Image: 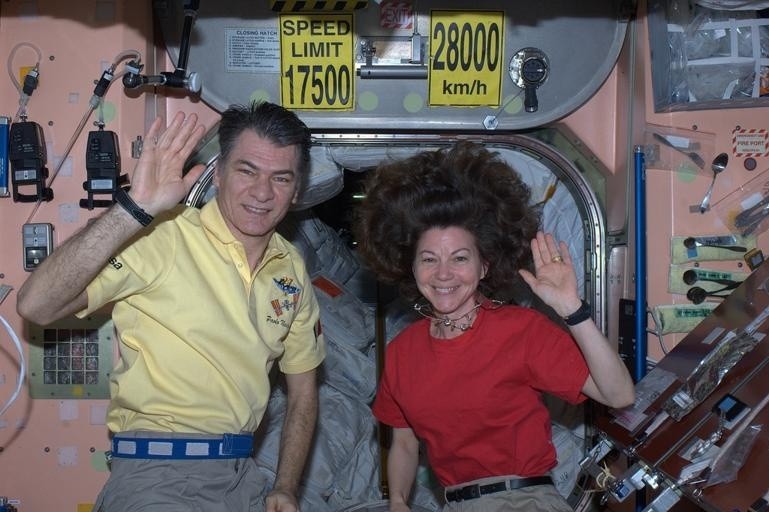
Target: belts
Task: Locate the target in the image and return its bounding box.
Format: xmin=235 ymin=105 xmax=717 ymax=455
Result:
xmin=110 ymin=432 xmax=256 ymax=461
xmin=444 ymin=475 xmax=554 ymax=504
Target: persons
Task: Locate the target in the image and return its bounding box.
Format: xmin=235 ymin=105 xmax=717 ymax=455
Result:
xmin=347 ymin=139 xmax=641 ymax=511
xmin=15 ymin=97 xmax=331 ymax=512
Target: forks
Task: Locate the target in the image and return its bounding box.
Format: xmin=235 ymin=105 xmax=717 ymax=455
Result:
xmin=654 ymin=133 xmax=706 ymax=169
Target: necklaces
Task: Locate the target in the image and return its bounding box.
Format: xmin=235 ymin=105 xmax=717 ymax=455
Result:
xmin=413 ymin=298 xmax=505 ymax=331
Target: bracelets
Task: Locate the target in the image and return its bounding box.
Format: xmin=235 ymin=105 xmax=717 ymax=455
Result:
xmin=560 ymin=298 xmax=592 ymax=326
xmin=113 ymin=189 xmax=154 ymax=229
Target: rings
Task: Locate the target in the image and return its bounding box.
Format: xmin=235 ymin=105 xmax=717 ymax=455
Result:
xmin=551 ymin=255 xmax=562 ymax=264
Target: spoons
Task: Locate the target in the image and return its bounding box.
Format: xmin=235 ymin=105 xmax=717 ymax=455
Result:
xmin=683 ymin=270 xmax=743 ymax=305
xmin=683 ymin=237 xmax=747 ymax=252
xmin=699 ymin=153 xmax=729 ymax=215
